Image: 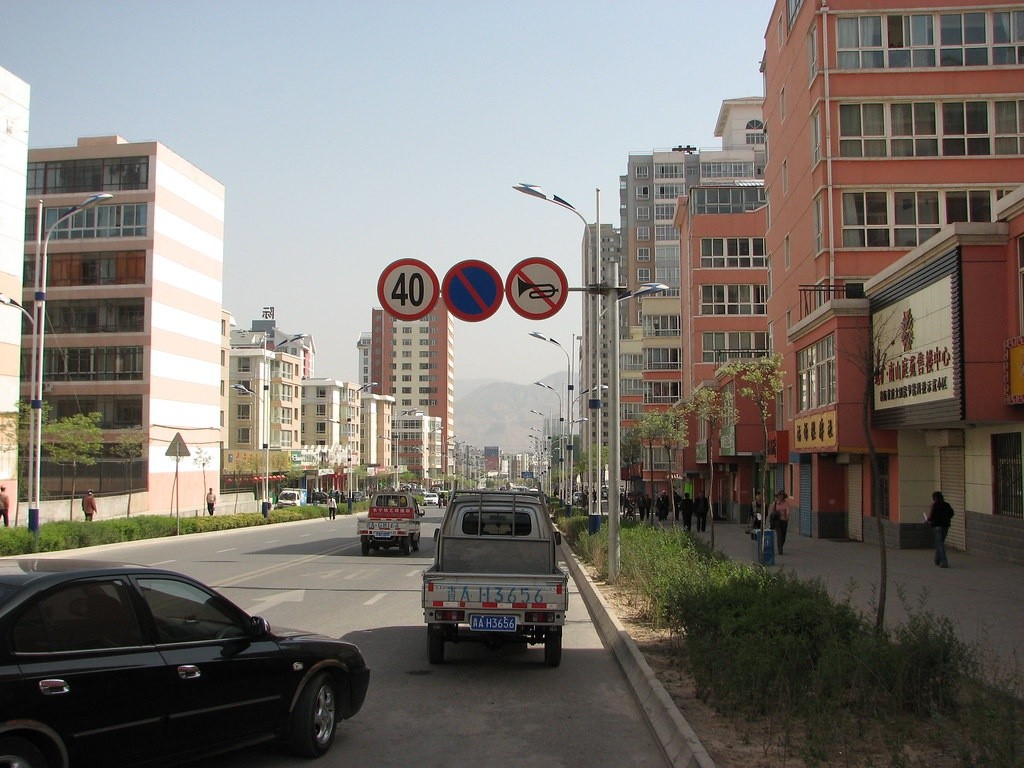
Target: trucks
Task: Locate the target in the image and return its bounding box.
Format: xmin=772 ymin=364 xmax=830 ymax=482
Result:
xmin=356 ymin=489 xmax=426 ymax=558
xmin=420 ymin=479 xmax=571 ymax=669
xmin=275 ymin=487 xmax=308 ymax=509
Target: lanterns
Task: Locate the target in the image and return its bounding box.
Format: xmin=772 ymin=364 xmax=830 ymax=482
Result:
xmin=226 ymin=475 xmax=285 ymax=484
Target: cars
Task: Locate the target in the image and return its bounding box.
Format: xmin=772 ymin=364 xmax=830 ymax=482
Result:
xmin=0 ymin=557 xmax=373 ymax=768
xmin=422 ymin=492 xmax=439 ymax=506
xmin=572 ymin=491 xmax=583 ymax=505
xmin=349 ymin=490 xmax=365 ymax=501
xmin=307 ymin=491 xmax=329 ymax=504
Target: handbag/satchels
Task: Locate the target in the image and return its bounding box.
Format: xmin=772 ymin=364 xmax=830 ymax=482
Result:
xmin=770 ymin=510 xmax=779 ymax=529
xmin=745 ymin=524 xmax=753 ymax=533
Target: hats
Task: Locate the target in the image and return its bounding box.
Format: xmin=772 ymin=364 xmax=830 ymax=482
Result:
xmin=775 ymin=489 xmax=786 ymax=498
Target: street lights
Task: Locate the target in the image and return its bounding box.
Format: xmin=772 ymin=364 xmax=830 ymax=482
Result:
xmin=0 ymin=193 xmax=118 ymax=536
xmin=229 ymin=333 xmax=310 ymax=517
xmin=324 ymin=381 xmax=379 ymax=514
xmin=526 ymin=427 xmax=562 ymax=495
xmin=377 ymin=408 xmax=418 ymax=493
xmin=512 ymin=182 xmax=671 ymax=535
xmin=529 ymin=408 xmax=568 ymax=505
xmin=527 ymin=330 xmax=609 ymax=518
xmin=533 ymin=379 xmax=589 ymax=507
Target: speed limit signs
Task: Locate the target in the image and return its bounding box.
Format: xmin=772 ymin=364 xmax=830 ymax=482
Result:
xmin=377 ymin=257 xmax=440 ymax=322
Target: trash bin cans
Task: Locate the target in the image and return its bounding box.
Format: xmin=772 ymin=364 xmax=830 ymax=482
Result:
xmin=751 ymin=529 xmax=775 ymax=566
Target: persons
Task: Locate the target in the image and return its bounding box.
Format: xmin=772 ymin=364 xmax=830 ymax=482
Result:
xmin=656 ymin=490 xmax=669 ymax=521
xmin=82 ymin=489 xmax=97 ymax=521
xmin=637 ymin=492 xmax=652 ymax=521
xmin=0 ymin=485 xmax=10 ymax=527
xmin=925 ymin=492 xmax=954 ymax=568
xmin=438 ymin=497 xmax=443 ymax=508
xmin=554 ymin=489 xmax=559 ymax=497
xmin=620 ymin=493 xmax=624 ymax=512
xmin=206 ymin=488 xmax=216 ymax=515
xmin=334 ymin=491 xmax=345 ymax=503
xmin=694 ymin=490 xmax=708 ymax=532
xmin=771 ymin=489 xmax=791 ymax=554
xmin=674 ymin=492 xmax=682 ymax=521
xmin=751 ymin=492 xmax=761 ymax=540
xmin=680 ymin=492 xmax=694 ymax=531
xmin=328 ymin=496 xmax=337 ymax=520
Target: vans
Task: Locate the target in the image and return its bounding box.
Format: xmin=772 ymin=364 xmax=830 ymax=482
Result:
xmin=601 ymin=484 xmax=625 ymax=499
